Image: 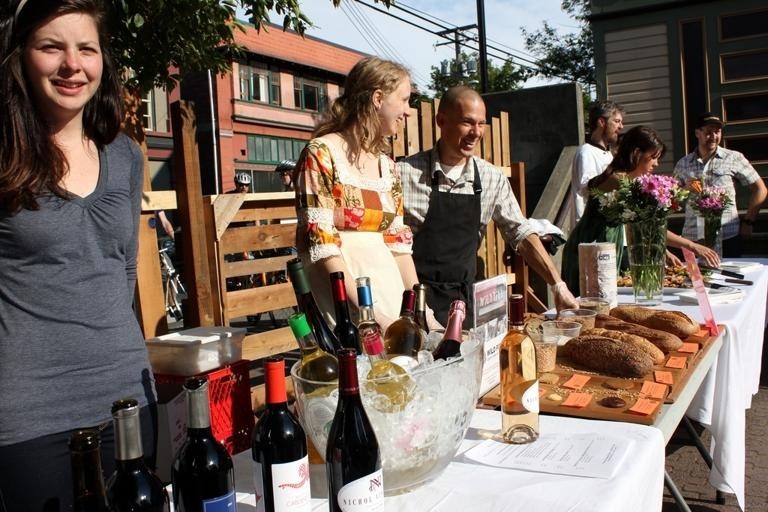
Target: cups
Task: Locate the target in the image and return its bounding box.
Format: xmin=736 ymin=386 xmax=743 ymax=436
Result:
xmin=527 ymin=298 xmax=610 ymax=372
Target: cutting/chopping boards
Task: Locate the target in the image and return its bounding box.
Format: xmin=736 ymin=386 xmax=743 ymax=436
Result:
xmin=482 ymin=322 xmax=725 ymax=425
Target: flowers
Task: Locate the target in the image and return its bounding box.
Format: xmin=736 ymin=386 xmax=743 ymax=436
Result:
xmin=581 ymin=170 xmax=690 ymax=300
xmin=680 ymin=174 xmax=734 ymax=258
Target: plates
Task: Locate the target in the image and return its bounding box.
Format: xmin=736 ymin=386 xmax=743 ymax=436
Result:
xmin=617 ymin=284 xmax=692 ymax=295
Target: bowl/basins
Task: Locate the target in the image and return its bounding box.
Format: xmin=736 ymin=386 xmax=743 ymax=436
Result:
xmin=287 ymin=331 xmax=483 ymax=498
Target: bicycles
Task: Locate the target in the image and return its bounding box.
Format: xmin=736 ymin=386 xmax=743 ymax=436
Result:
xmin=159 ymin=242 xmax=188 ymax=323
xmin=234 ymin=225 xmax=299 ymax=331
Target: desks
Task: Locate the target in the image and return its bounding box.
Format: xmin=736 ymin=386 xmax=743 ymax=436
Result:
xmin=539 ymin=251 xmax=768 ymax=506
xmin=167 ymin=310 xmax=728 ymax=512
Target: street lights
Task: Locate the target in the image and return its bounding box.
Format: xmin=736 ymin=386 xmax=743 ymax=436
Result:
xmin=438 ymin=56 xmax=480 ymax=91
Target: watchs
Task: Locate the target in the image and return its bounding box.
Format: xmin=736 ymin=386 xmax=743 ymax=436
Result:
xmin=744 ymin=217 xmax=755 ymax=227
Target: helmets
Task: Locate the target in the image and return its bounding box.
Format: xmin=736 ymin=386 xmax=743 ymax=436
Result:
xmin=234 ymin=170 xmax=252 ymax=185
xmin=274 ymin=159 xmax=297 ymax=172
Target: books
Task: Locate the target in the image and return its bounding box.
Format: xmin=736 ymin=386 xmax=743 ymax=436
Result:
xmin=681 ymin=287 xmax=744 ymax=303
xmin=719 ymin=260 xmax=763 ymax=271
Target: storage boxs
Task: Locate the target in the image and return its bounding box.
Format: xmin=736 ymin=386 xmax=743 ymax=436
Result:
xmin=152 ymin=361 xmax=257 ymax=459
xmin=139 ymin=323 xmax=249 ymax=375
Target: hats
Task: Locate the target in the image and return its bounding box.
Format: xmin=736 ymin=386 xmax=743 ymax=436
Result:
xmin=695 ymin=112 xmax=724 ymax=129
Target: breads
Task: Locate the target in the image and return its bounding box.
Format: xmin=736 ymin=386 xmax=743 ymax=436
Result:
xmin=564 ymin=306 xmax=701 ymax=378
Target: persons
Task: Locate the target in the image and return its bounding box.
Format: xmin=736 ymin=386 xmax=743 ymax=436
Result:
xmin=221 ymin=171 xmax=266 ymax=289
xmin=273 ymin=158 xmax=300 ymax=282
xmin=404 ymin=86 xmax=584 ymax=328
xmin=558 ymin=125 xmax=721 ymax=297
xmin=1 ymin=2 xmax=161 ymax=509
xmin=156 ymin=208 xmax=176 ymax=318
xmin=569 ymin=99 xmax=625 ymax=235
xmin=671 ymin=112 xmax=768 ymax=258
xmin=292 ymin=57 xmax=444 ymax=338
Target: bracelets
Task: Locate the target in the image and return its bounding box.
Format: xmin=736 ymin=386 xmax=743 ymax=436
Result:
xmin=551 ymin=281 xmax=570 ymax=295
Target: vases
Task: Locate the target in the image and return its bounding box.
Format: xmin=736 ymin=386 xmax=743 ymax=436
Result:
xmin=620 ymin=212 xmax=669 ymax=305
xmin=703 ymin=215 xmax=722 ymax=267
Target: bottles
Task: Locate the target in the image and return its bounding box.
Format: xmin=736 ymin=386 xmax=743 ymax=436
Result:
xmin=324 ymin=349 xmax=386 ymax=511
xmin=104 ymin=397 xmax=171 ymax=511
xmin=499 ymin=294 xmax=541 ymax=445
xmin=172 ymin=378 xmax=236 ymax=510
xmin=250 ymin=358 xmax=313 ymax=512
xmin=285 ymin=256 xmax=469 ymax=466
xmin=71 ymin=431 xmax=110 ymax=505
